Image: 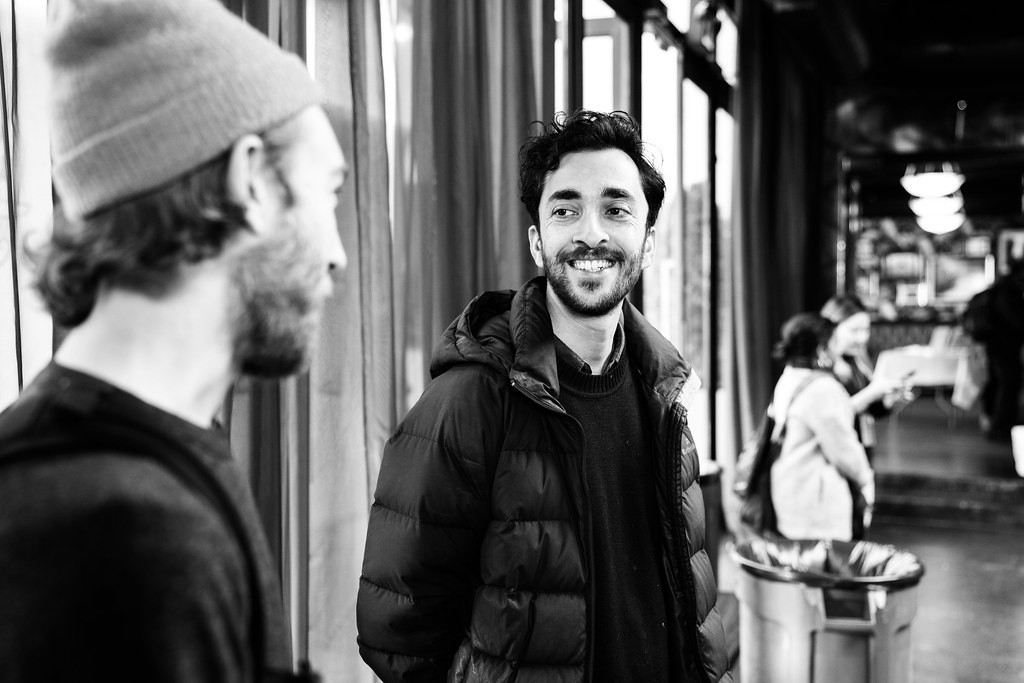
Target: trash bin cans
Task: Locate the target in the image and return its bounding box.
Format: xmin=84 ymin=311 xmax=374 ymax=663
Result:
xmin=734 ymin=539 xmax=925 ymax=683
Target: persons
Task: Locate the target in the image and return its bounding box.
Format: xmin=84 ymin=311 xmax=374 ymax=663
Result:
xmin=766 ymin=314 xmax=878 ymax=543
xmin=353 ymin=110 xmax=738 ymax=683
xmin=0 ymin=1 xmax=348 ymax=683
xmin=817 ymin=292 xmax=917 ymax=542
xmin=964 ymin=256 xmax=1023 ymax=442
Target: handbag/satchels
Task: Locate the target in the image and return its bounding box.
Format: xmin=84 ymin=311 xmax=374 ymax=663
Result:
xmin=731 ymin=410 xmax=776 ymax=503
xmin=739 ymin=443 xmax=782 ymax=538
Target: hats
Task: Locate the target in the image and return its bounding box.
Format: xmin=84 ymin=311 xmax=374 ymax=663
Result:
xmin=47 ymin=0 xmax=324 ymax=221
